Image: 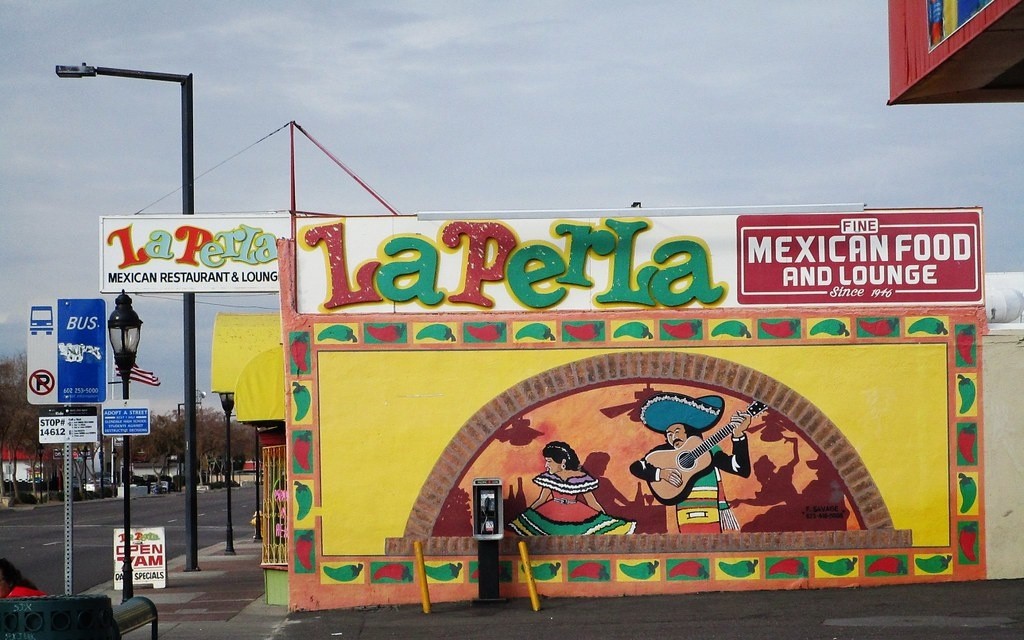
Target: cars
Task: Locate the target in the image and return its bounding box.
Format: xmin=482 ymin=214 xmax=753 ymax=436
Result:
xmin=5 ymin=471 xmax=172 ymax=497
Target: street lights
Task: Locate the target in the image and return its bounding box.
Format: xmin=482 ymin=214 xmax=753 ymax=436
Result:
xmin=108 ymin=289 xmax=144 ymax=606
xmin=217 ymin=392 xmax=236 ymax=557
xmin=56 ymin=60 xmax=198 ymax=570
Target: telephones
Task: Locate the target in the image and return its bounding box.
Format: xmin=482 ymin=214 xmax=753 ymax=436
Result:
xmin=481 ymin=497 xmax=495 ymax=511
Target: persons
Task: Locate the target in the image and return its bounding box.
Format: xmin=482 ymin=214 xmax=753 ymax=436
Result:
xmin=0 ymin=557 xmax=47 ymax=598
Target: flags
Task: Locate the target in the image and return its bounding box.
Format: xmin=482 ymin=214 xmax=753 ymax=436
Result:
xmin=113 ymin=352 xmax=161 ymax=386
